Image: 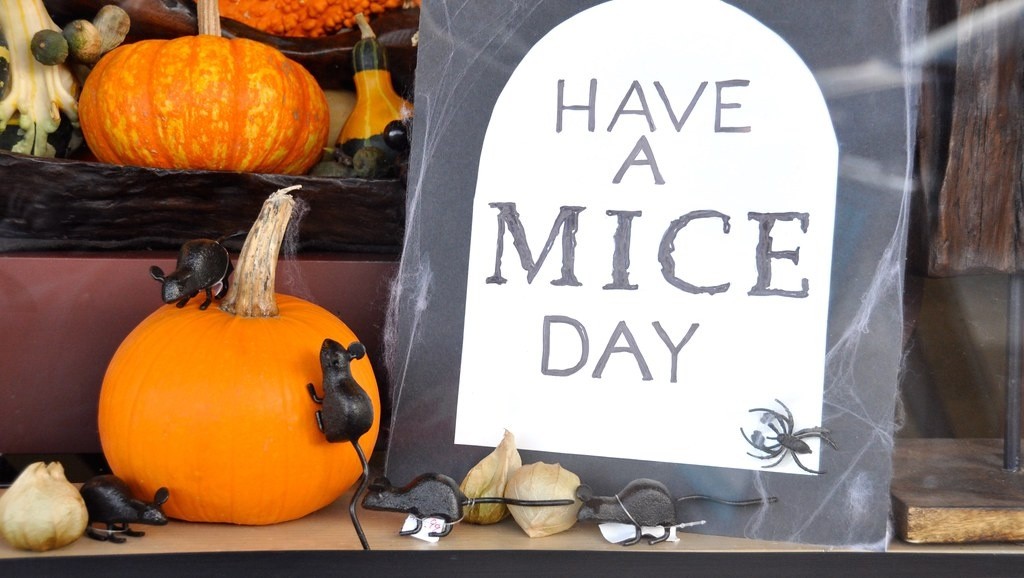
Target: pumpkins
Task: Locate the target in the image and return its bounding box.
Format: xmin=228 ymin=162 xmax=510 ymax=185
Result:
xmin=97 ymin=184 xmax=380 ymax=526
xmin=0 ymin=0 xmax=423 ymax=177
